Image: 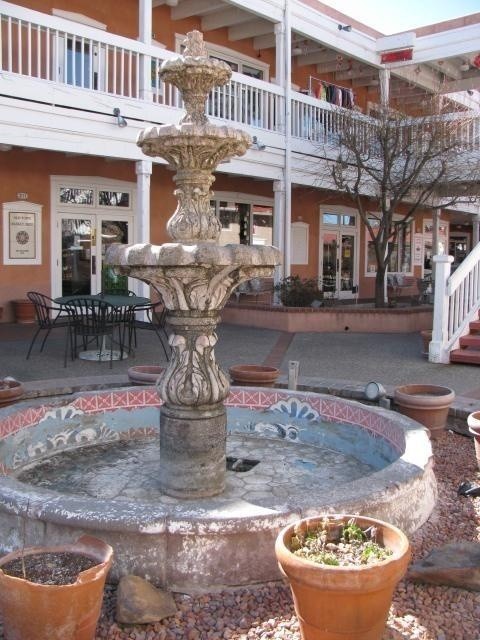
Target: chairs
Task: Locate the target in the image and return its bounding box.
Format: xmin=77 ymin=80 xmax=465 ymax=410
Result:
xmin=21 ymin=286 xmax=176 ymax=368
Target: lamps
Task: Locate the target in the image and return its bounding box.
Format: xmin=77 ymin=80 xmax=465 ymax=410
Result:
xmin=363 ymin=380 xmax=393 ymax=410
xmin=249 ymin=133 xmax=269 ymax=154
xmin=109 ymin=105 xmax=131 ymax=130
xmin=336 ymin=20 xmax=354 ymax=36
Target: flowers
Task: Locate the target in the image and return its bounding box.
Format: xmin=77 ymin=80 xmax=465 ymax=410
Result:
xmin=271 ymin=272 xmax=321 ymax=294
xmin=287 ymin=512 xmax=388 ymax=567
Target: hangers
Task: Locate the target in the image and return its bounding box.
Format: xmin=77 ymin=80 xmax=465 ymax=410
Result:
xmin=314 ymin=79 xmax=354 ymax=92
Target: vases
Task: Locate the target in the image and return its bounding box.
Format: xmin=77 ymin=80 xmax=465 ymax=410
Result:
xmin=279 ymin=287 xmax=315 ymax=308
xmin=12 ymin=298 xmax=39 ymax=327
xmin=0 ymin=378 xmax=27 ymax=411
xmin=228 ymin=365 xmax=281 ymax=389
xmin=0 ymin=533 xmax=117 ymax=640
xmin=466 ymin=409 xmax=480 ymax=471
xmin=391 ymin=382 xmax=457 ymax=440
xmin=127 ymin=363 xmax=168 ymax=387
xmin=273 ymin=511 xmax=413 ymax=640
